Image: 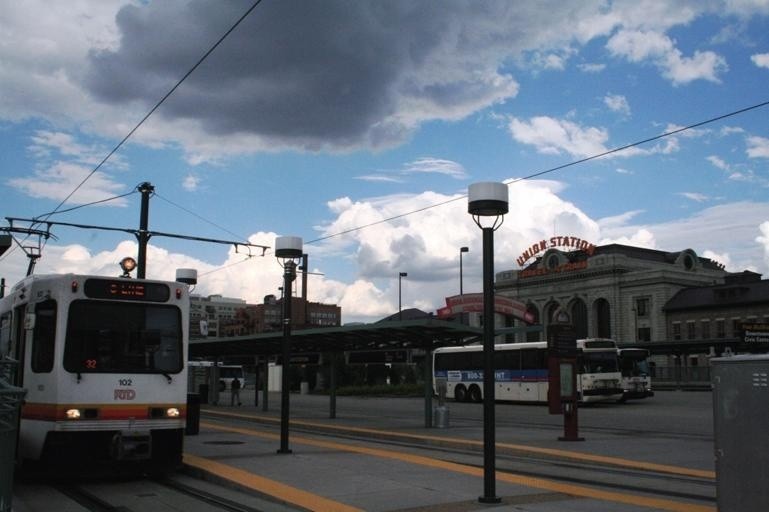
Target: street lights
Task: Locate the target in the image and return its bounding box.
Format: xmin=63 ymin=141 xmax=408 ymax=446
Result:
xmin=399 ymin=272 xmax=407 ymax=321
xmin=460 ymin=246 xmax=469 ymax=295
xmin=275 ymin=235 xmax=302 ymax=453
xmin=467 ymin=182 xmax=509 ymax=505
xmin=278 ymin=287 xmax=283 ymax=330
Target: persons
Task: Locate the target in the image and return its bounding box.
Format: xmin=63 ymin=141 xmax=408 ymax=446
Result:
xmin=230 ymin=376 xmax=242 ymax=407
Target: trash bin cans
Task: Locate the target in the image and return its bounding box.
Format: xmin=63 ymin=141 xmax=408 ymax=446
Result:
xmin=434 ymin=406 xmax=449 ymax=428
xmin=186 ymin=392 xmax=200 ymax=434
xmin=199 ymin=384 xmax=208 ymax=404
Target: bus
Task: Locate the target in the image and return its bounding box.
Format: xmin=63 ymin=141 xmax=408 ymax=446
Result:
xmin=431 ymin=337 xmax=654 ymax=405
xmin=188 ymin=360 xmax=247 ymax=392
xmin=0 ymin=258 xmax=189 ymax=476
